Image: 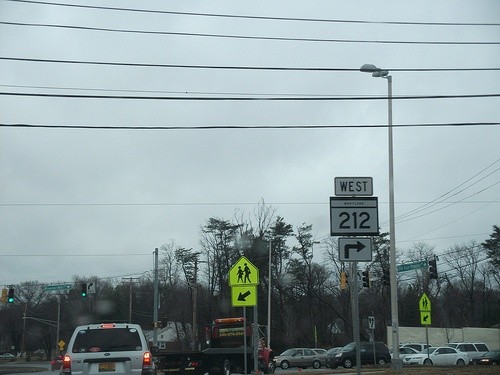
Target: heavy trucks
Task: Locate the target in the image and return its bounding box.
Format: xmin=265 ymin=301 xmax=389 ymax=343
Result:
xmin=153 ymin=317 xmax=276 ymax=375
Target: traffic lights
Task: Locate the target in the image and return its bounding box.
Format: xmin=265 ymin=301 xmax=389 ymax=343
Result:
xmin=81 ymin=284 xmax=87 ymax=297
xmin=429 ymin=259 xmax=437 ymax=278
xmin=9 ymin=289 xmax=14 ymax=303
xmin=362 ymin=271 xmax=371 ymax=288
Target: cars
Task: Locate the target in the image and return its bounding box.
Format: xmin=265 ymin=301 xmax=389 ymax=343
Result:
xmin=398 ymin=343 xmax=432 ymax=351
xmin=446 ymin=342 xmax=491 ymax=361
xmin=315 ymin=347 xmax=342 ymax=368
xmin=399 ymin=347 xmax=420 ymax=361
xmin=469 ymin=350 xmax=500 ymax=366
xmin=404 ymin=347 xmax=469 ymax=367
xmin=274 ymin=348 xmax=326 ymax=370
xmin=51 ymin=356 xmax=63 ymax=371
xmin=325 ymin=341 xmax=391 ymax=368
xmin=59 ymin=323 xmax=157 ymax=375
xmin=0 ymin=353 xmax=14 ymax=360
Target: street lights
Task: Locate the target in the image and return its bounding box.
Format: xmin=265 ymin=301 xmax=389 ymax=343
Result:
xmin=359 ymin=64 xmax=399 ymax=358
